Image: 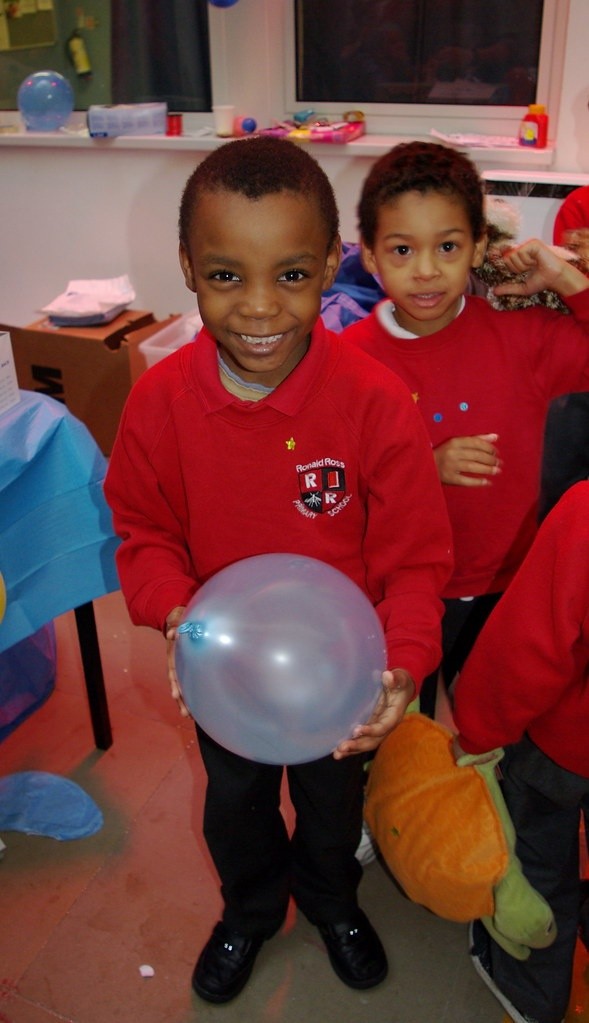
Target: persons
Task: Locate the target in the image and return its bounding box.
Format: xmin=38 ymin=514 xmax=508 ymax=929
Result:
xmin=102 ymin=136 xmax=456 ymax=1002
xmin=553 ymin=186 xmax=589 ymax=248
xmin=453 ymin=480 xmax=589 ymax=1023
xmin=342 ymin=142 xmax=588 ymax=868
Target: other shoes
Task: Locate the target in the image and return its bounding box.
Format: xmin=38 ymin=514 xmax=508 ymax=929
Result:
xmin=468 ymin=919 xmax=561 ymax=1023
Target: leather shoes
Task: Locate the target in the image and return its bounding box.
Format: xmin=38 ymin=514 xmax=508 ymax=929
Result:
xmin=191 ymin=920 xmax=284 ymax=1006
xmin=318 ymin=905 xmax=389 ymax=990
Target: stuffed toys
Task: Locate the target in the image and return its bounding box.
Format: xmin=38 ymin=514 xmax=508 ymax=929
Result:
xmin=473 ymin=198 xmax=568 ymax=314
xmin=364 ymin=696 xmax=557 ymax=959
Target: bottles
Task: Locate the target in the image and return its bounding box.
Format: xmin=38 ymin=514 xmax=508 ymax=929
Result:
xmin=519 ymin=104 xmax=549 ymax=149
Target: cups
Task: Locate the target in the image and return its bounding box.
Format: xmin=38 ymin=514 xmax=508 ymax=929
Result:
xmin=212 ymin=105 xmax=235 ymax=138
xmin=166 ymin=114 xmax=183 ymax=137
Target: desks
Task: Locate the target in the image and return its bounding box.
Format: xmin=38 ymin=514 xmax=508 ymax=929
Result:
xmin=0 ymin=391 xmax=112 ymax=752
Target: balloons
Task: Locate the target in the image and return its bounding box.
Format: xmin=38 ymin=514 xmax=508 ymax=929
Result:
xmin=17 ymin=71 xmax=75 ymax=132
xmin=174 ymin=553 xmax=386 ymax=762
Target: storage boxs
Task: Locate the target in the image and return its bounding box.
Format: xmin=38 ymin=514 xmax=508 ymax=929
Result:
xmin=87 ymin=103 xmax=167 ymax=138
xmin=0 ymin=307 xmax=204 ymax=457
